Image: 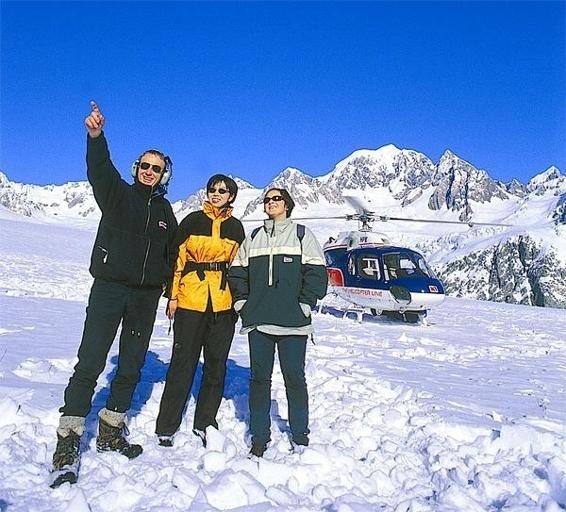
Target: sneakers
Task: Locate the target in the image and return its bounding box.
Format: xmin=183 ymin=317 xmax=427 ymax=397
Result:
xmin=249 ymin=443 xmax=269 ymax=459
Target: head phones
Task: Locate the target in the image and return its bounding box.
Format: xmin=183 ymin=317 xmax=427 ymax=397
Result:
xmin=131 ymin=150 xmax=173 ymax=186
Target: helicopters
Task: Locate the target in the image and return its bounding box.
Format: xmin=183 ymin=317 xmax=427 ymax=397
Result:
xmin=239 ymin=208 xmax=513 ymax=327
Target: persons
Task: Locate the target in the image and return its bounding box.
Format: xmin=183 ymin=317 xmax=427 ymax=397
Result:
xmin=226 ymin=187 xmax=328 ymax=463
xmin=154 ymin=173 xmax=246 ymax=454
xmin=46 ymin=99 xmax=179 ymax=492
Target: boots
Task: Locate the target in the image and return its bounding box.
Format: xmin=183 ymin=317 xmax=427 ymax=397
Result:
xmin=94 ymin=405 xmax=142 ymax=461
xmin=46 ymin=414 xmax=88 ymax=489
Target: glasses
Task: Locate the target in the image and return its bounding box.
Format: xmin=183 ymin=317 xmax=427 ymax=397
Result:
xmin=263 ymin=196 xmax=283 ymax=204
xmin=138 ymin=162 xmax=164 ymax=174
xmin=207 ymin=187 xmax=230 ymax=195
